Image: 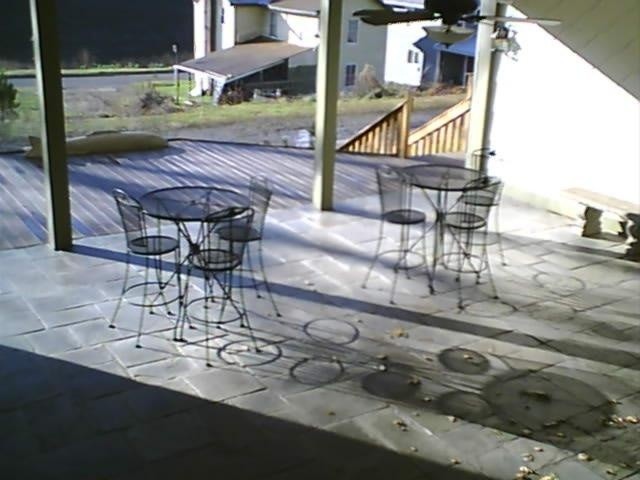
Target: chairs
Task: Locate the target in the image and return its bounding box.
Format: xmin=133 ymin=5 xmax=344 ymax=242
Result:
xmin=106 ymin=174 xmax=285 ymax=369
xmin=361 ymin=165 xmax=508 ymax=301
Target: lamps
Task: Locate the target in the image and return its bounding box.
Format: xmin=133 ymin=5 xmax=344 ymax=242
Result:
xmin=420 ymin=1 xmax=481 ymax=48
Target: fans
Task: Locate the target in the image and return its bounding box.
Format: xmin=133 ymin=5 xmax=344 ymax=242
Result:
xmin=352 ymin=1 xmax=563 ymax=49
